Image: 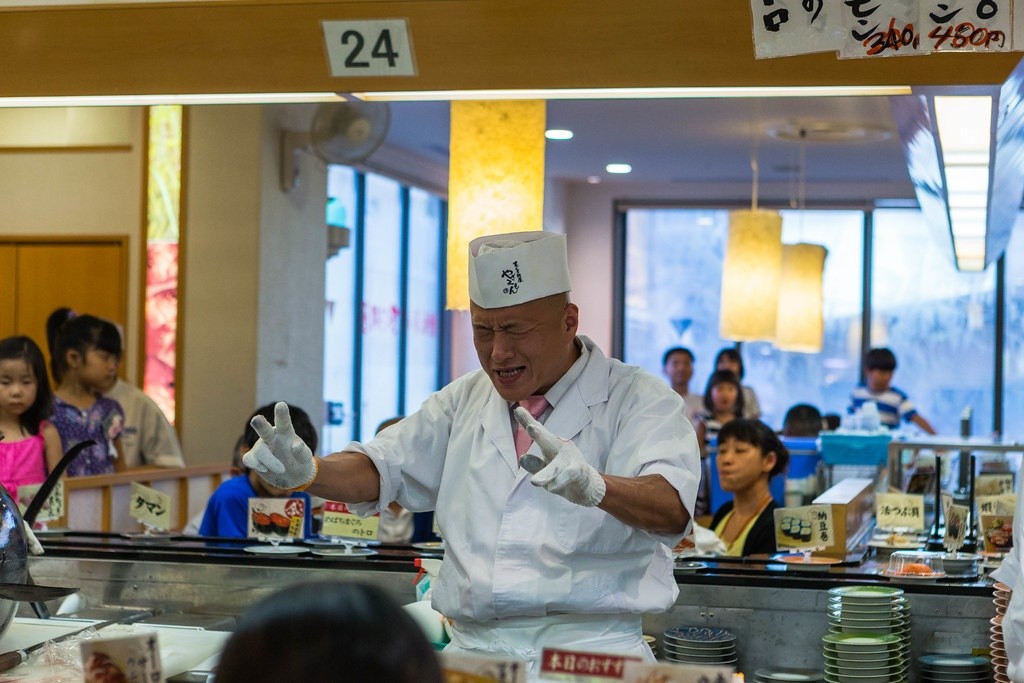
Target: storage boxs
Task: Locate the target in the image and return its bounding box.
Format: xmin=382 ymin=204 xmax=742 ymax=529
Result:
xmin=817 ymin=429 xmax=912 ymax=467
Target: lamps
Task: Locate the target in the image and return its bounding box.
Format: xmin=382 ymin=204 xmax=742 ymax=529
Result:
xmin=717 ymin=98 xmax=782 ymax=340
xmin=444 ymin=96 xmax=547 ymax=315
xmin=775 ymin=126 xmax=828 ymax=353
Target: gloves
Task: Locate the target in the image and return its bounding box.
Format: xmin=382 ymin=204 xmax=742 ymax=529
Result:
xmin=241 ymin=401 xmax=320 ymax=493
xmin=513 ymin=405 xmax=607 ymax=508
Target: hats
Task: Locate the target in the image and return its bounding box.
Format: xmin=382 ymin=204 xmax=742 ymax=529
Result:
xmin=468 ymin=230 xmax=571 ymax=310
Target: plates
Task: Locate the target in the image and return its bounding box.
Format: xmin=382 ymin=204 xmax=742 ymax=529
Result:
xmin=775 ymin=556 xmax=843 ymax=571
xmin=412 ymin=542 xmax=445 ymax=551
xmin=243 ymin=546 xmax=309 ymax=557
xmin=929 ymin=556 xmax=983 ymax=568
xmin=672 ymin=562 xmax=707 ymax=575
xmin=989 ymin=582 xmax=1012 ymax=683
xmin=642 ymin=626 xmax=737 ymax=664
xmin=878 ymin=570 xmax=947 ymax=585
xmin=918 ymin=654 xmax=993 ymax=683
xmin=867 ymin=540 xmax=926 ymax=553
xmin=820 ymin=585 xmax=912 ymax=683
xmin=752 ymin=669 xmax=824 ymax=683
xmin=310 ymin=546 xmax=380 ymax=561
xmin=304 ymin=539 xmax=381 ymax=548
xmin=874 ymin=534 xmax=928 ymax=542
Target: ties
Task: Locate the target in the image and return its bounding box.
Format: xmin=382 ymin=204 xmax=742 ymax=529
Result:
xmin=514 ymin=394 xmax=549 ymax=469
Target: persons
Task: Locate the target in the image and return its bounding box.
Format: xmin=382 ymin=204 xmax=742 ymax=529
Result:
xmin=989 ymin=457 xmax=1024 ymax=683
xmin=708 ymin=417 xmax=789 ymax=557
xmin=0 ymin=335 xmax=64 ymax=527
xmin=92 ymin=322 xmax=186 ymax=469
xmin=215 ymin=578 xmax=449 ymax=682
xmin=662 ymin=346 xmax=935 ymax=463
xmin=182 ymin=402 xmax=443 ymax=545
xmin=241 ymin=230 xmax=702 ymax=671
xmin=42 ymin=307 xmax=129 ymax=477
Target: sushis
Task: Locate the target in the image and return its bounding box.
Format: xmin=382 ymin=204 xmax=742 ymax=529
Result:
xmin=780 ymin=516 xmax=812 ymax=544
xmin=85 ymin=652 xmax=127 ymax=683
xmin=252 ymin=512 xmax=292 ymax=538
xmin=896 ymin=564 xmax=934 ymax=575
xmin=985 ymin=518 xmax=1013 ymax=547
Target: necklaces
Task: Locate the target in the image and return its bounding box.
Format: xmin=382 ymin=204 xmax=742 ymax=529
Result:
xmin=719 ymin=493 xmax=771 ymax=550
